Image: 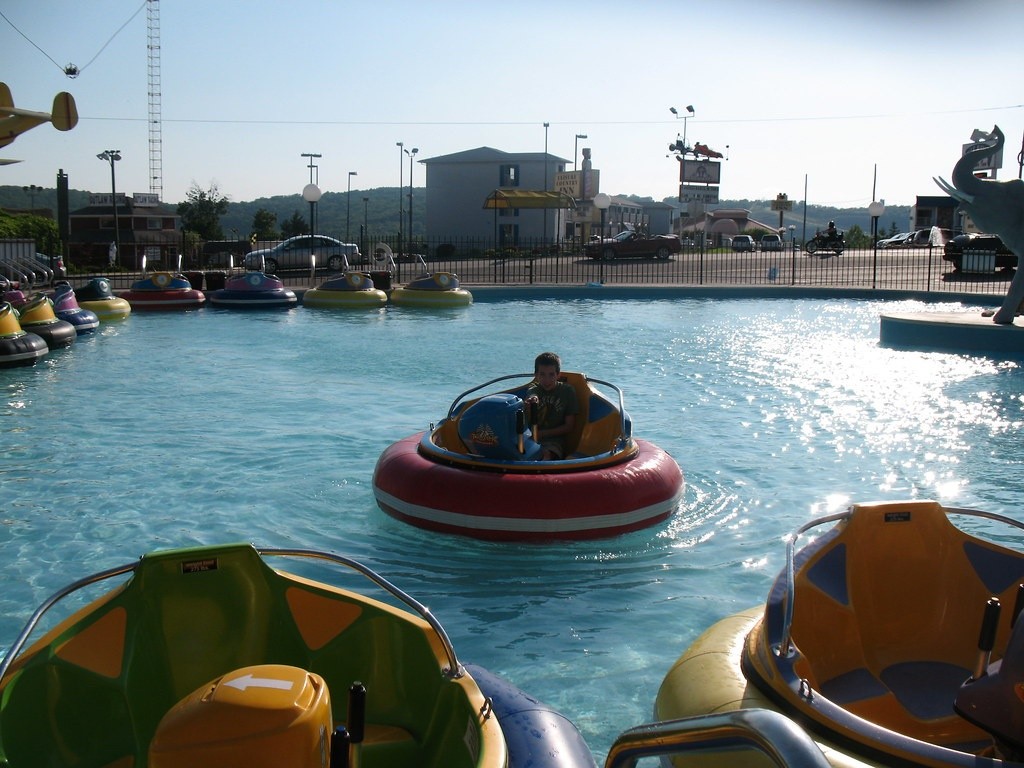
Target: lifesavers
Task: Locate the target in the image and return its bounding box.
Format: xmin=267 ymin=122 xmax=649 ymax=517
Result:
xmin=369 ymin=242 xmax=393 ymax=267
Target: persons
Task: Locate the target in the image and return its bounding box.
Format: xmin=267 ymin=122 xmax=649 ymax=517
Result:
xmin=820 ymin=221 xmax=837 ymax=247
xmin=524 ymin=352 xmax=586 ymax=460
xmin=631 ymin=232 xmax=645 ymax=241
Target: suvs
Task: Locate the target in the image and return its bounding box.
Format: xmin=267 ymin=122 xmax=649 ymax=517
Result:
xmin=732 ymin=235 xmax=756 ymax=252
xmin=760 ymin=234 xmax=783 ymax=251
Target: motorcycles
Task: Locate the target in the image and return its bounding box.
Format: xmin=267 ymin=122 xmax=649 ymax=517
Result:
xmin=805 ymin=232 xmax=846 ymax=254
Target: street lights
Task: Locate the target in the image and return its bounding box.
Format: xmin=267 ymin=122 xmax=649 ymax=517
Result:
xmin=669 ymin=105 xmax=695 ymax=235
xmin=543 ymin=122 xmax=549 ymax=256
xmin=301 ymin=153 xmax=322 ymax=256
xmin=396 ymin=143 xmax=418 ymax=261
xmin=97 ymin=150 xmax=121 ymax=271
xmin=346 ymin=171 xmax=368 ymax=243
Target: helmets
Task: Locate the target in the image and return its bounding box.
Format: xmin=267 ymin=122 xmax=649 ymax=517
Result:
xmin=828 ymin=221 xmax=835 ymax=227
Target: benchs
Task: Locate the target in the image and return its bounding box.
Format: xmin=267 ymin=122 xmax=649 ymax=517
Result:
xmin=0 ymin=543 xmax=507 ymax=768
xmin=764 ymin=501 xmax=1024 ymax=747
xmin=445 ymin=372 xmax=632 ymax=457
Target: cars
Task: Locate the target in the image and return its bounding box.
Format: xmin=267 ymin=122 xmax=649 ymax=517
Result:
xmin=876 ymin=229 xmax=967 ymax=250
xmin=583 ymin=231 xmax=681 ymax=261
xmin=943 ymin=232 xmax=1018 ymax=271
xmin=243 ymin=234 xmax=362 ymax=274
xmin=36 ymin=252 xmax=65 ymax=273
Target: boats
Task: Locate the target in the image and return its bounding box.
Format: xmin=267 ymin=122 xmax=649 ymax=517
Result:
xmin=128 ymin=272 xmax=473 ymax=311
xmin=1 ymin=544 xmax=596 ymax=768
xmin=653 ymin=504 xmax=1024 ymax=768
xmin=372 ymin=372 xmax=683 ymax=538
xmin=0 ymin=279 xmax=131 ymax=367
